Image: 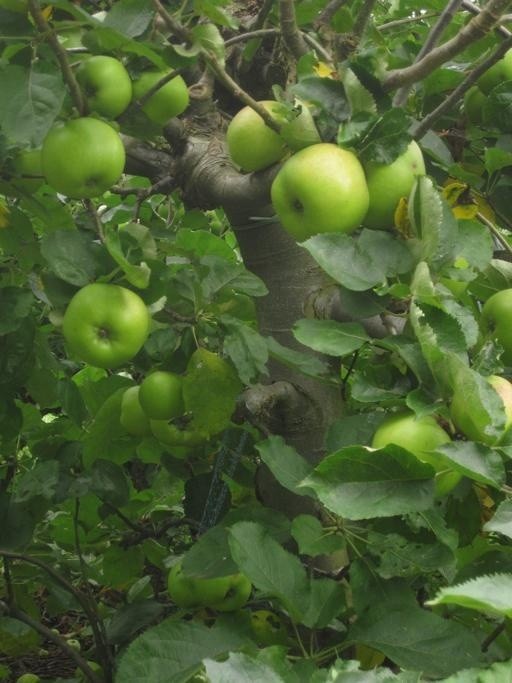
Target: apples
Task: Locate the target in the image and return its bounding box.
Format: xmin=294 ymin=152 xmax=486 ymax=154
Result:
xmin=486 ymin=374 xmax=512 ymax=428
xmin=249 ymin=610 xmax=286 ymax=646
xmin=75 ymin=661 xmax=103 ymax=683
xmin=170 ymin=551 xmax=252 ymax=611
xmin=17 ymin=674 xmax=40 ymax=683
xmin=227 ymin=99 xmax=425 ymax=244
xmin=373 ymin=413 xmax=462 ymax=499
xmin=50 ymin=629 xmax=59 ymax=636
xmin=478 ymin=290 xmax=512 ymax=363
xmin=120 ymin=373 xmax=183 ymax=438
xmin=40 ymin=53 xmax=191 ymax=201
xmin=67 ymin=639 xmax=81 ymax=653
xmin=64 ymin=280 xmax=152 ymax=368
